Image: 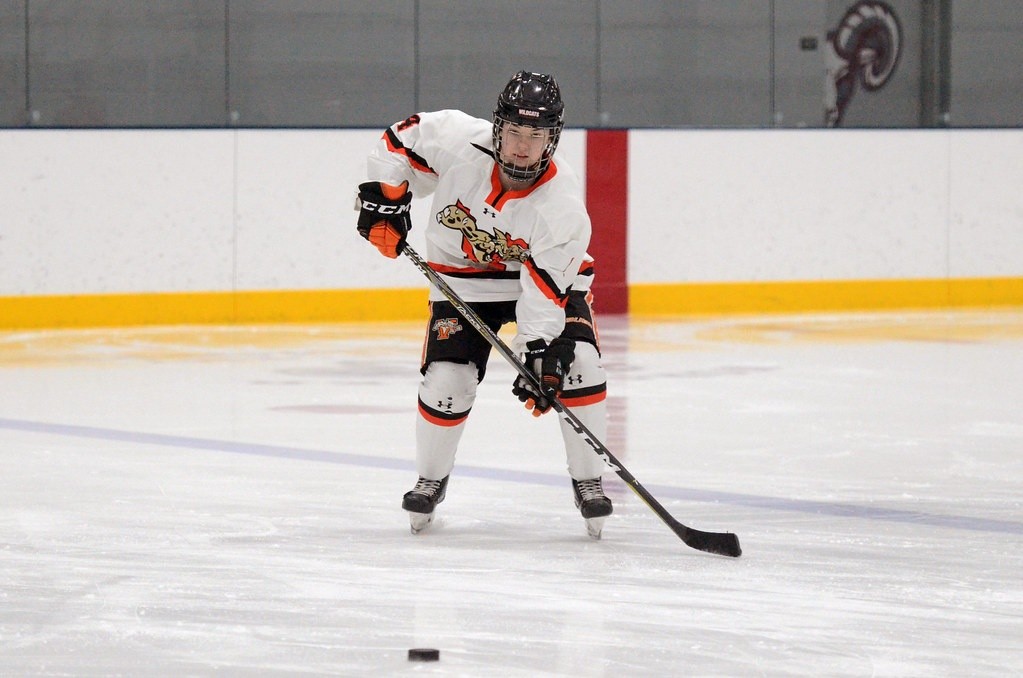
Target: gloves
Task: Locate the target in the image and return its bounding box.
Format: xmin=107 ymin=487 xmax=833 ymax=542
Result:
xmin=355 ymin=181 xmax=413 ymax=259
xmin=513 ymin=335 xmax=575 ymax=417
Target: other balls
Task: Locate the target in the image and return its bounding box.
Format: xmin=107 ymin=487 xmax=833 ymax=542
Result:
xmin=408 ymin=648 xmax=439 ymax=662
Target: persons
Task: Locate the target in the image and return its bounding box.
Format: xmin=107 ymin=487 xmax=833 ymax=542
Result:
xmin=354 ymin=71 xmax=613 ymax=540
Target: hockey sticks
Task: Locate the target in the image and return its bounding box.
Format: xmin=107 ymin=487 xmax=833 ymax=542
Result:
xmin=400 ymin=240 xmax=743 ymax=560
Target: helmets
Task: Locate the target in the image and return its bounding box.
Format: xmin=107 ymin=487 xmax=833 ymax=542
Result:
xmin=492 ymin=72 xmax=564 ymax=182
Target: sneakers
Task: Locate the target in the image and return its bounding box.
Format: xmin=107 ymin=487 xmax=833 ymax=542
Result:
xmin=402 ymin=476 xmax=448 ymax=534
xmin=572 ymin=476 xmax=612 ymax=540
